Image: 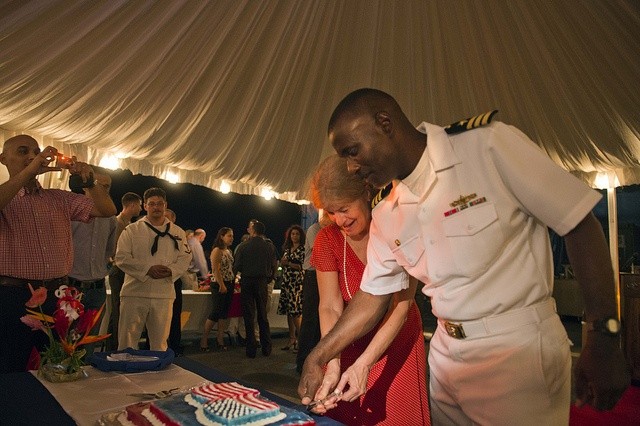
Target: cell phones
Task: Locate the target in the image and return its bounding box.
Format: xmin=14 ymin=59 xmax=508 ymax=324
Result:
xmin=56 ymin=153 xmax=72 ymax=169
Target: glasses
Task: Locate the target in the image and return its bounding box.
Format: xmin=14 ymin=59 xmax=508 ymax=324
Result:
xmin=146 ymin=201 xmax=166 ymax=206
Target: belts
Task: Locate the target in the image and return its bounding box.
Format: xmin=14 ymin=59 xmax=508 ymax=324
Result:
xmin=440 ymin=301 xmax=555 ymax=339
xmin=2 ymin=276 xmax=67 ymax=291
xmin=70 ymin=277 xmax=107 ymax=289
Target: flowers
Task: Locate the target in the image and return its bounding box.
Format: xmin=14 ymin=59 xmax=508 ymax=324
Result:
xmin=22 ymin=283 xmax=110 ymax=376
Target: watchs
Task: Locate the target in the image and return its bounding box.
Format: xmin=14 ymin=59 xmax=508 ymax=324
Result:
xmin=580 ymin=314 xmax=627 ymax=336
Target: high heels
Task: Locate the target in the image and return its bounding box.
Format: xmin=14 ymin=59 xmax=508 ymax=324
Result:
xmin=281 ymin=337 xmax=298 ymax=350
xmin=290 ymin=339 xmax=299 ymax=354
xmin=199 ymin=341 xmax=211 ymax=353
xmin=215 ymin=339 xmax=228 ymax=352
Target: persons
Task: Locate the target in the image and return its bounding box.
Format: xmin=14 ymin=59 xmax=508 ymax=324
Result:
xmin=295 ymin=221 xmax=322 ymax=374
xmin=233 ymin=222 xmax=277 ymax=358
xmin=65 ymin=173 xmax=117 ymax=365
xmin=164 ymin=207 xmax=177 ymax=222
xmin=187 ymin=227 xmax=209 ymax=289
xmin=241 ymin=218 xmax=257 ymax=242
xmin=199 ymin=227 xmax=235 ymax=352
xmin=0 ymin=134 xmax=117 ymax=399
xmin=114 ymin=188 xmax=194 ymax=352
xmin=276 ymin=225 xmax=304 ymax=352
xmin=104 ymin=191 xmax=142 ymax=353
xmin=311 ymin=153 xmax=431 ymax=426
xmin=327 ymin=90 xmax=631 ymax=426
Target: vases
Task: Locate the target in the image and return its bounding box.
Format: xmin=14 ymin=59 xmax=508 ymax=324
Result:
xmin=40 ymin=364 xmax=85 ymax=383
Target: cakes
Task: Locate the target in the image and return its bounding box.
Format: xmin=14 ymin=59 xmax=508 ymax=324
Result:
xmin=98 ymin=380 xmax=342 ymax=426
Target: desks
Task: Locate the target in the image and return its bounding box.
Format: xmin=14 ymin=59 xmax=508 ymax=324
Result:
xmin=1 ymin=351 xmax=349 ymax=426
xmin=101 ymin=289 xmax=290 ymax=344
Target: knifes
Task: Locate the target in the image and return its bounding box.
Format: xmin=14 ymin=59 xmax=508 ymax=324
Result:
xmin=290 ymin=390 xmax=341 ymax=412
